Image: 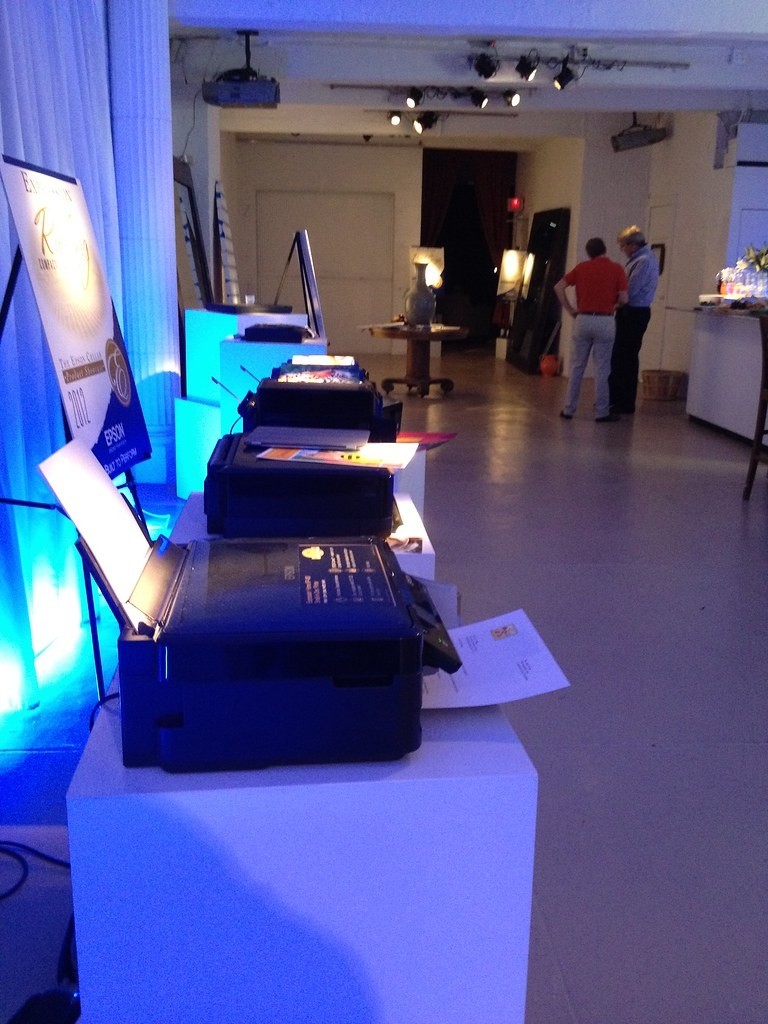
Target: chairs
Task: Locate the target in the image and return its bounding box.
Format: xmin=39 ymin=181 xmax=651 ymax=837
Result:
xmin=743 ymin=317 xmax=768 ymax=501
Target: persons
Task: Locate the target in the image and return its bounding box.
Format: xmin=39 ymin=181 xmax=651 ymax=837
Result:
xmin=554 ymin=237 xmax=629 ymax=423
xmin=594 ymin=224 xmax=659 ymax=414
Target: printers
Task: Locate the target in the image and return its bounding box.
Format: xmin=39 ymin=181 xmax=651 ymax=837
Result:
xmin=202 ymin=434 xmax=394 ymax=541
xmin=75 ymin=507 xmax=462 ymax=773
xmin=238 ymin=378 xmax=403 ymax=441
xmin=271 ymin=359 xmax=365 ymax=381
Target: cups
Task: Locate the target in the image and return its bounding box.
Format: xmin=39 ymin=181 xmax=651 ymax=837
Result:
xmin=245 ymin=295 xmax=255 ymax=305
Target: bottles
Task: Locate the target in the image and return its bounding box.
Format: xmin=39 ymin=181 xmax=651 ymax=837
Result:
xmin=405 ymin=263 xmax=436 ymax=329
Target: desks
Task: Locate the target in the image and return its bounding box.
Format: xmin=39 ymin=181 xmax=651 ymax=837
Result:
xmin=65 ymin=310 xmax=540 ymax=1024
xmin=370 ymin=324 xmax=460 ymax=399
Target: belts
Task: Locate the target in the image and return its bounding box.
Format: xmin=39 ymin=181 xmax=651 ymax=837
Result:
xmin=585 ymin=312 xmax=611 ymax=316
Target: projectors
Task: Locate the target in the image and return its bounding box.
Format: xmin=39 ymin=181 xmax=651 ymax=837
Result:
xmin=201 ymin=80 xmax=281 ymax=108
xmin=611 ymin=128 xmax=666 ymax=152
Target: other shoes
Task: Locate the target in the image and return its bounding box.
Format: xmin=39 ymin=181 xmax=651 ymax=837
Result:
xmin=595 ymin=413 xmax=620 ymax=422
xmin=609 ymin=405 xmax=635 ymax=413
xmin=561 ymin=410 xmax=572 ymax=419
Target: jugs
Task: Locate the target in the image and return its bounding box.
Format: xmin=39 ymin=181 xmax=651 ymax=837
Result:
xmin=715 ymin=269 xmax=768 ymax=296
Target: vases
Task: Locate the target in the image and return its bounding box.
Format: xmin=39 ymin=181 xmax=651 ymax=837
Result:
xmin=405 ymin=263 xmax=436 ymax=326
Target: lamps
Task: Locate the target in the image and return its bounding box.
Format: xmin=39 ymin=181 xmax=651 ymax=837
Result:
xmin=471 ymin=91 xmax=488 ymax=109
xmin=515 ymin=55 xmax=537 ymax=82
xmin=409 ymin=245 xmax=444 ymax=291
xmin=406 ymin=87 xmax=423 ymax=108
xmin=553 ymin=56 xmax=575 ymax=91
xmin=503 ymin=90 xmax=521 ymax=107
xmin=474 ymin=54 xmax=498 ymax=79
xmin=389 ymin=111 xmax=401 ymax=125
xmin=497 ymin=247 xmax=527 ymax=301
xmin=414 ymin=111 xmax=437 ymax=134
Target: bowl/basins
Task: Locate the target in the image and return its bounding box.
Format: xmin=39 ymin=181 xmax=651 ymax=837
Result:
xmin=699 ymin=295 xmax=723 ymax=306
